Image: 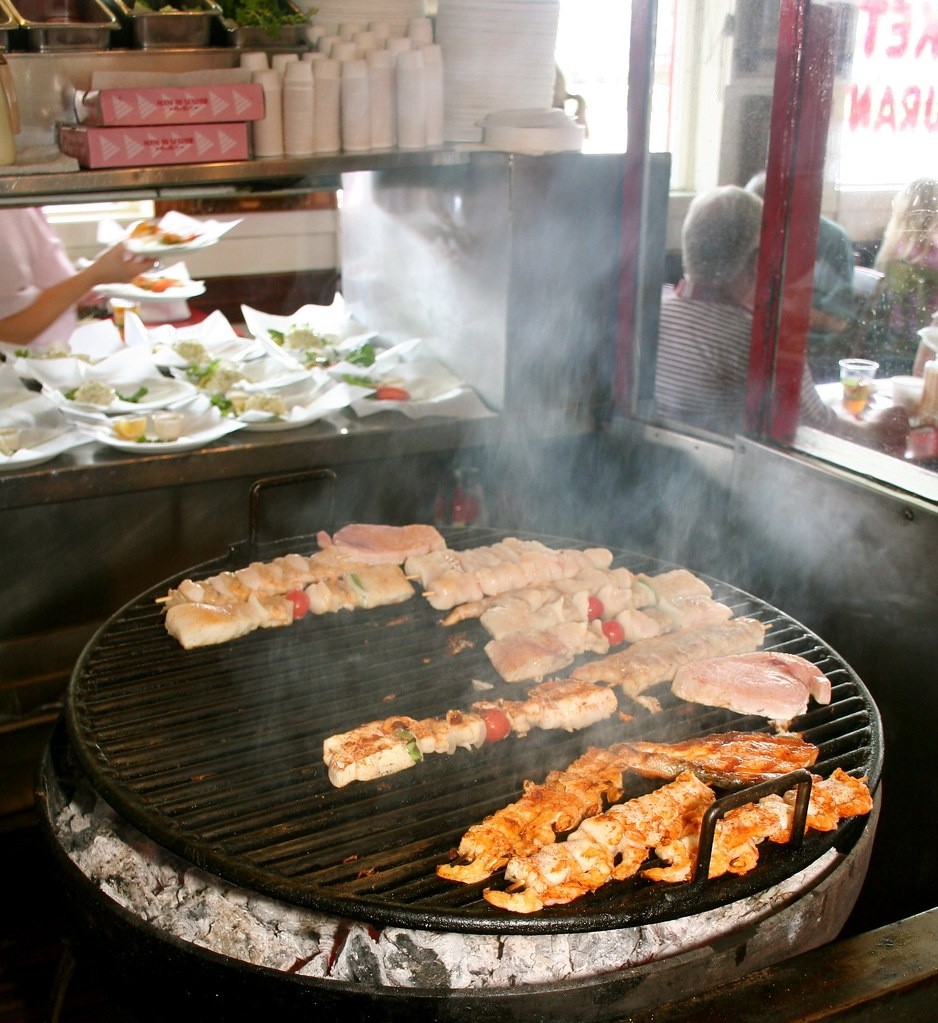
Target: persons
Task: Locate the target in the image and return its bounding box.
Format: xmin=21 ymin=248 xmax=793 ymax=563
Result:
xmin=0 ymin=204 xmax=160 ymax=344
xmin=873 ymin=178 xmax=938 ymax=346
xmin=652 ymin=174 xmax=909 ymax=446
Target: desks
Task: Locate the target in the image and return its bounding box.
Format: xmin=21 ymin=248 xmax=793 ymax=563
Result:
xmin=815 ymin=375 xmax=925 ymax=429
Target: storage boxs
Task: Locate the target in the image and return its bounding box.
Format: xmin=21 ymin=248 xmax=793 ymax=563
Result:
xmin=75 ymin=82 xmax=266 ymax=128
xmin=58 ymin=120 xmax=254 ymax=169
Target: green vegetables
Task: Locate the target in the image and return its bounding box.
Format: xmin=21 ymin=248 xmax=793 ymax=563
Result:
xmin=119 ymin=385 xmax=149 ymax=404
xmin=231 ymin=0 xmax=319 ymax=42
xmin=64 ymin=389 xmax=76 ymax=400
xmin=14 ymin=349 xmax=32 ymax=358
xmin=187 ymin=329 xmax=379 ymax=412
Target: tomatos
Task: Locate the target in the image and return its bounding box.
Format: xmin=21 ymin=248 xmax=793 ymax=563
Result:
xmin=589 ymin=596 xmax=604 ymax=620
xmin=602 ymin=620 xmax=627 ymax=645
xmin=284 ymin=590 xmax=310 ymax=620
xmin=480 ymin=709 xmax=509 ymax=742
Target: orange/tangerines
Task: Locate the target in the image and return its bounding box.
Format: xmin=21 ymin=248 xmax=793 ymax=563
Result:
xmin=114 ymin=419 xmax=146 ymax=439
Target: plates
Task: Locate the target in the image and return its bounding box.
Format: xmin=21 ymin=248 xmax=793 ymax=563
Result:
xmin=98 ymin=209 xmax=243 ymax=255
xmin=0 ymin=257 xmax=469 ymax=471
xmin=433 ymin=0 xmax=585 ymax=154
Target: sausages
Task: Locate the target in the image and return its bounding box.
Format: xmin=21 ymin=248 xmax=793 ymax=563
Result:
xmin=378 ymin=388 xmax=411 ymax=400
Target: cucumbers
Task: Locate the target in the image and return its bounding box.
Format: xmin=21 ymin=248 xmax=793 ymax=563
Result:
xmin=401 ymin=730 xmax=421 ymax=761
xmin=638 ymin=579 xmax=659 ymax=606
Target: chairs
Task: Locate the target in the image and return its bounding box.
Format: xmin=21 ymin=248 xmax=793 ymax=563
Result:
xmin=840 ymin=264 xmax=885 ymax=357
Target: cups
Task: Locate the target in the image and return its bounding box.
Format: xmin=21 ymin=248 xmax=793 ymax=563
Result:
xmin=915 ymin=360 xmax=938 ymax=429
xmin=240 ymin=14 xmax=444 ymax=159
xmin=839 ymin=358 xmax=880 ymax=414
xmin=891 ymin=376 xmax=923 ymax=416
